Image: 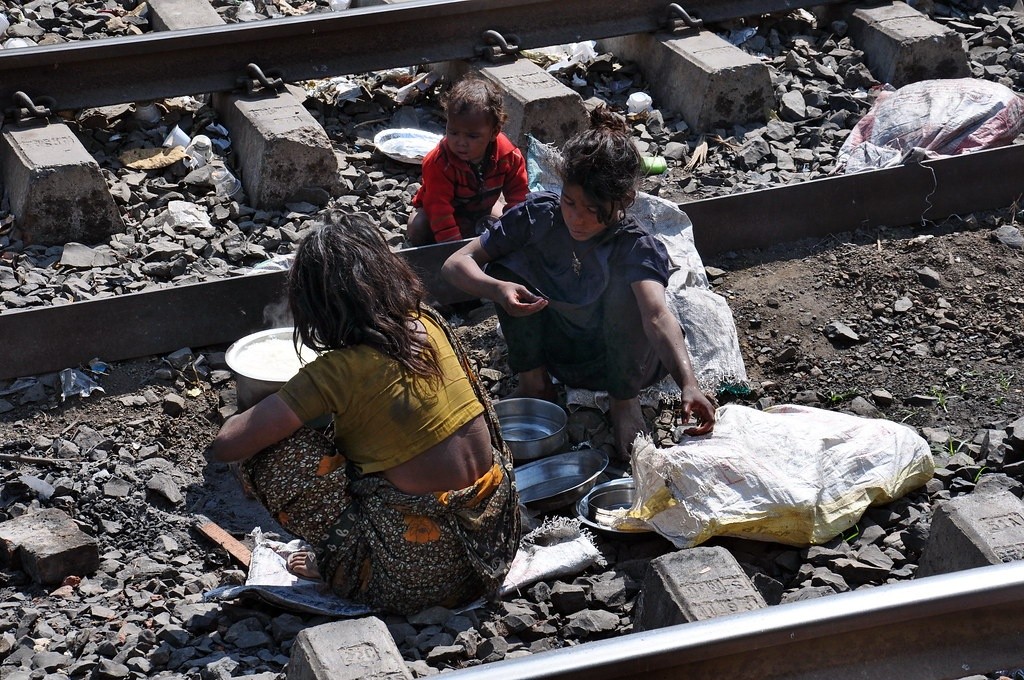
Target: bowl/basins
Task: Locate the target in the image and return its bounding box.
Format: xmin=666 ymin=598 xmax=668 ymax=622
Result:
xmin=570 ymin=477 xmax=654 ymax=543
xmin=493 ymin=398 xmax=567 ymax=461
xmin=513 ymin=449 xmax=609 ymax=513
xmin=224 ymin=326 xmax=334 ymax=430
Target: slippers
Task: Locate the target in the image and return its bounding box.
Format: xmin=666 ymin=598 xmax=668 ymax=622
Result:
xmin=287 ymin=552 xmax=325 ymax=583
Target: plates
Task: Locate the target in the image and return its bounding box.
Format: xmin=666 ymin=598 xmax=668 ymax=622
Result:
xmin=372 ymin=128 xmax=440 ymax=164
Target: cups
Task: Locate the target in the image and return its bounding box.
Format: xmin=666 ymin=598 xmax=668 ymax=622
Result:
xmin=642 ymin=157 xmax=666 ymax=175
xmin=212 ymin=169 xmax=243 ymax=197
xmin=134 ymin=101 xmax=162 ymax=125
xmin=160 ymin=125 xmax=191 ymax=152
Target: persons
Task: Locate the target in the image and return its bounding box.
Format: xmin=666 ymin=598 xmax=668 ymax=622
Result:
xmin=214 ymin=213 xmax=522 ymax=616
xmin=442 ymin=103 xmax=717 ymax=461
xmin=407 ymin=78 xmax=533 ymax=247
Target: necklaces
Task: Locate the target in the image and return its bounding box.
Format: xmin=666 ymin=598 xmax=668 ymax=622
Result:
xmin=569 ymin=236 xmax=601 ymax=277
